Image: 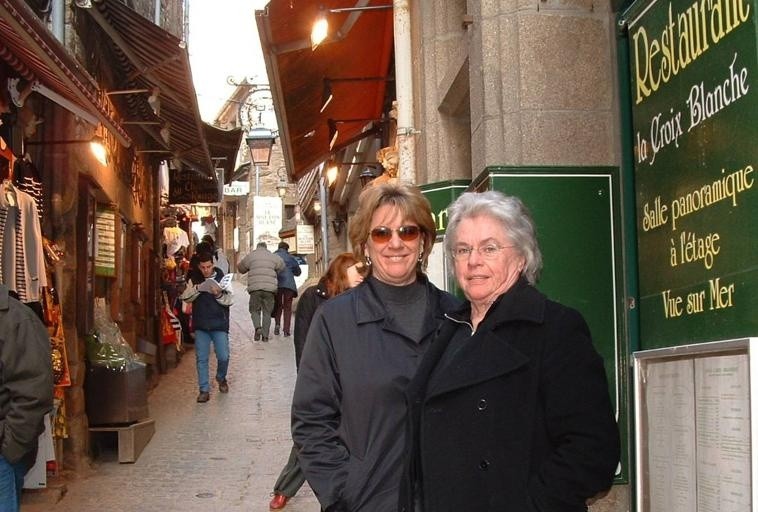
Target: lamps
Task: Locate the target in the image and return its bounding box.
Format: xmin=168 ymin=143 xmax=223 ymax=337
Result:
xmin=326 ymin=160 xmax=380 ymax=187
xmin=327 ymin=117 xmax=389 ymax=151
xmin=244 ymin=120 xmax=276 ymax=168
xmin=119 ymin=120 xmax=173 ymax=148
xmin=108 ymin=88 xmax=163 ymax=117
xmin=25 ymin=134 xmax=109 ymax=166
xmin=136 ymin=149 xmax=183 ymax=174
xmin=307 ymin=3 xmax=397 ymax=53
xmin=318 ymin=76 xmax=395 ymax=113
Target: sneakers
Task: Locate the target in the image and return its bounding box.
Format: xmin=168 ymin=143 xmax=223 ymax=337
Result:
xmin=215 ymin=375 xmax=228 ymax=393
xmin=284 ymin=332 xmax=291 ymax=337
xmin=274 ymin=325 xmax=280 ymax=335
xmin=270 ymin=494 xmax=291 ymax=512
xmin=262 ymin=336 xmax=269 ymax=342
xmin=253 ymin=327 xmax=262 ymax=341
xmin=196 ymin=391 xmax=210 ymax=403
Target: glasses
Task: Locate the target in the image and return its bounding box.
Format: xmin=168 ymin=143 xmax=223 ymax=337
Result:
xmin=365 ymin=224 xmax=422 ymax=244
xmin=451 ymin=240 xmax=518 ymax=262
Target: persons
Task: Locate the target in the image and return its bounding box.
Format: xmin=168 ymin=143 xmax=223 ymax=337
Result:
xmin=1 ymin=279 xmax=57 ymax=512
xmin=396 ymin=188 xmax=622 ymax=511
xmin=179 ymin=233 xmax=300 ymax=404
xmin=268 ymin=249 xmax=367 ymax=510
xmin=288 ymin=178 xmax=467 ymax=512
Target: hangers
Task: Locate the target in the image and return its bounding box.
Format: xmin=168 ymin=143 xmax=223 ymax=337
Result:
xmin=2 ymin=179 xmax=17 ymax=207
